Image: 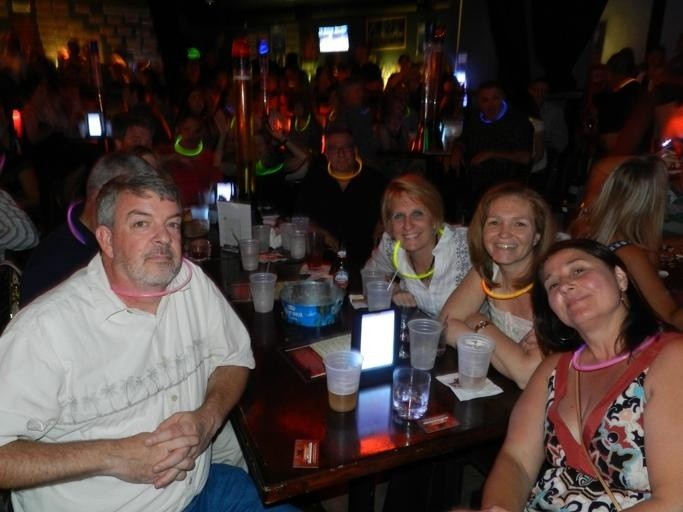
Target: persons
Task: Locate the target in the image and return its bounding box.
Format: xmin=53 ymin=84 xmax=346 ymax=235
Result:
xmin=0 ymin=172 xmax=263 ymax=510
xmin=481 ymin=238 xmax=683 ymax=511
xmin=437 ymin=182 xmax=554 ymax=511
xmin=0 ymin=0 xmax=683 ymax=395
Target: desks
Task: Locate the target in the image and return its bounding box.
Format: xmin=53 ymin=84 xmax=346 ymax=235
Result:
xmin=149 ymin=204 xmax=523 ymax=511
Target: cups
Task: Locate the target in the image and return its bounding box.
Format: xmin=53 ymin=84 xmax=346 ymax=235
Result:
xmin=322 ymin=349 xmax=364 ymax=411
xmin=237 ymin=238 xmax=260 ymax=271
xmin=253 ymin=215 xmax=324 ymax=261
xmin=359 ymin=266 xmax=395 ymax=311
xmin=456 ymin=331 xmax=496 ymax=393
xmin=408 ymin=317 xmax=439 ymax=372
xmin=391 ymin=367 xmax=432 ymax=421
xmin=183 ymin=219 xmax=210 ymax=262
xmin=249 ymin=271 xmax=277 ymax=314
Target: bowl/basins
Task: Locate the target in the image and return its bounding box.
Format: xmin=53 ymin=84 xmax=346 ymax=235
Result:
xmin=280 ymin=281 xmax=345 ymax=328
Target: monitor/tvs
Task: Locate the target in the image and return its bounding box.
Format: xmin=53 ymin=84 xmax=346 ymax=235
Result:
xmin=314 ymin=20 xmax=351 ymax=54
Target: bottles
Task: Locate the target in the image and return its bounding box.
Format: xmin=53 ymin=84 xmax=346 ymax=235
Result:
xmin=333 ymin=242 xmax=351 ymax=296
